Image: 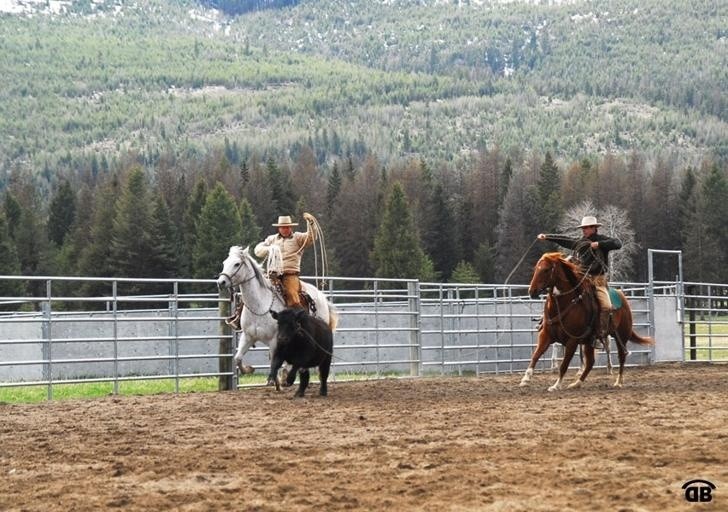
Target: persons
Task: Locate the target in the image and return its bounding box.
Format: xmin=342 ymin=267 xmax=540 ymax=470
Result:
xmin=253 ymin=211 xmax=320 ymax=315
xmin=536 ymin=215 xmax=625 ymax=338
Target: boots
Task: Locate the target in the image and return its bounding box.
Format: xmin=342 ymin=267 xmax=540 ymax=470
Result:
xmin=595 ymin=309 xmax=610 ymax=338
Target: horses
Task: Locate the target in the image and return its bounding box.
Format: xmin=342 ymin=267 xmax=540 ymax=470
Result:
xmin=216 ymin=245 xmax=338 ymax=394
xmin=518 ymin=251 xmax=655 ymax=394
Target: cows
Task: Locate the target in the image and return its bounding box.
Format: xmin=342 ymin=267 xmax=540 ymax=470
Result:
xmin=269 ymin=308 xmax=333 ymax=397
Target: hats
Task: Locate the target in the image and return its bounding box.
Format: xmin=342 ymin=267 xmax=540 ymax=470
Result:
xmin=576 ymin=216 xmax=604 ymax=228
xmin=269 ymin=215 xmax=300 ymax=227
